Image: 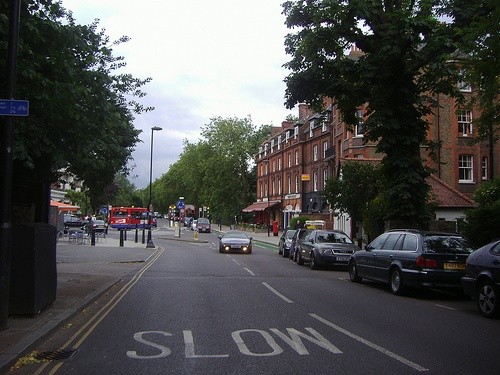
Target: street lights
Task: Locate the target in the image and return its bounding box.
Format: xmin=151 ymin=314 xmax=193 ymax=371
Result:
xmin=146 ymin=125 xmax=163 ymax=247
xmin=262 ymin=160 xmax=271 ymax=237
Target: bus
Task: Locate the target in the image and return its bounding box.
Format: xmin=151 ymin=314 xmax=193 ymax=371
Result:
xmin=109 ymin=207 xmax=158 ymax=229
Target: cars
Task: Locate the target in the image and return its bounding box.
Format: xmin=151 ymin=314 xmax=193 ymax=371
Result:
xmin=86 ymin=220 xmax=109 ymax=234
xmin=154 ymin=212 xmax=211 ymax=233
xmin=63 ymin=214 xmax=83 ymax=226
xmin=347 ymin=228 xmax=475 ymax=302
xmin=460 ymin=239 xmax=500 ymax=320
xmin=278 ymin=227 xmax=361 ymax=272
xmin=217 ymin=230 xmax=253 ymax=254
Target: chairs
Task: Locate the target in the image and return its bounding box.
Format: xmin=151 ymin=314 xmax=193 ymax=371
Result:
xmin=57 ymin=230 xmax=65 ymax=242
xmin=68 ymin=229 xmax=106 ymax=246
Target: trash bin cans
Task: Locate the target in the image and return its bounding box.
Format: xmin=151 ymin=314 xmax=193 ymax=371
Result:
xmin=273 ymin=221 xmax=279 ymax=236
